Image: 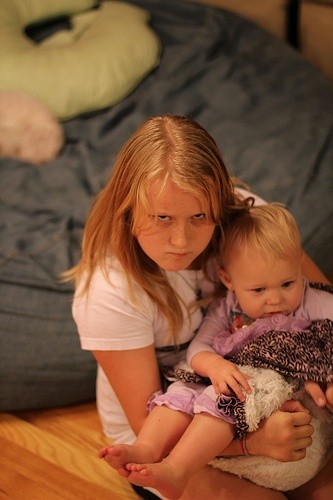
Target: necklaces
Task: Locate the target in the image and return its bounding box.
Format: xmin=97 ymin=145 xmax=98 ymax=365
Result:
xmin=177 ymin=263 xmax=206 ymax=320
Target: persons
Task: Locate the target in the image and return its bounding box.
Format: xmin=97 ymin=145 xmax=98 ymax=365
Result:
xmin=98 ymin=202 xmax=333 ymax=500
xmin=59 ymin=116 xmax=333 ymax=500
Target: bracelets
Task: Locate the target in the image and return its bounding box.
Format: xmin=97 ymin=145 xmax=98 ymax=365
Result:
xmin=242 ymin=432 xmax=250 ymax=455
